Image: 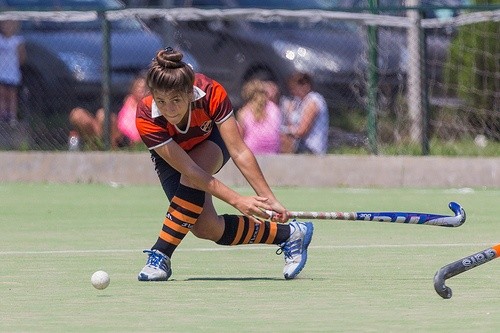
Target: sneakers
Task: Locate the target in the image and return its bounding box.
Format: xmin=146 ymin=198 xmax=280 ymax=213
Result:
xmin=138 ymin=249 xmax=173 ymax=281
xmin=276 ymin=219 xmax=313 ymax=280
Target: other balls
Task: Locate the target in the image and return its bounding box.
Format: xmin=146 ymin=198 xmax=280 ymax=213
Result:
xmin=91 ymin=271 xmax=110 ymax=290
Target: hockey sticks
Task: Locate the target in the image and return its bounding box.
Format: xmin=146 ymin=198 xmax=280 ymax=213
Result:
xmin=432 ymin=245 xmax=500 ymax=299
xmin=256 ymin=202 xmax=467 ymax=227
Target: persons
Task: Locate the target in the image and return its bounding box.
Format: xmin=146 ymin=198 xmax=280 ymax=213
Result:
xmin=135 ymin=48 xmax=314 ymax=280
xmin=0 ymin=19 xmax=329 ymax=155
